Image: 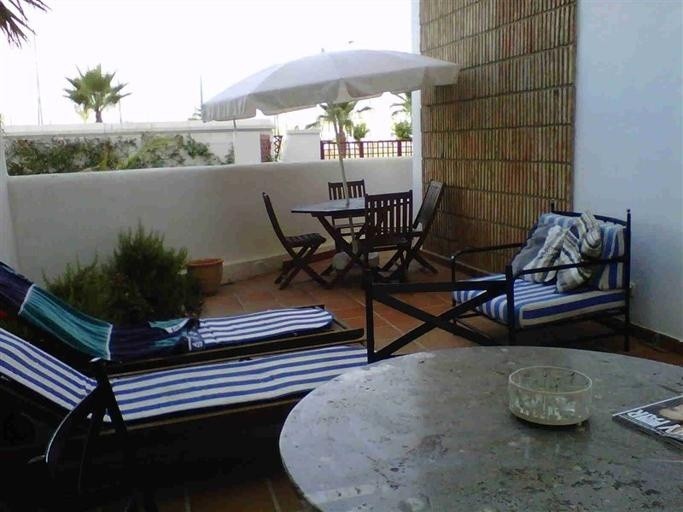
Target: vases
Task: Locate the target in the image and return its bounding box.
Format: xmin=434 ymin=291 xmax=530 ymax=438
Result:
xmin=185 ymin=259 xmax=223 ymax=297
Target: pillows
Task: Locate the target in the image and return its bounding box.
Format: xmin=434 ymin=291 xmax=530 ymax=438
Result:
xmin=556 ymin=208 xmax=602 ymax=292
xmin=510 ymin=224 xmax=571 ymax=287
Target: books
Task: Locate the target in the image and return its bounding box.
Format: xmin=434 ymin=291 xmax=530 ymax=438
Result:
xmin=612 ymin=395 xmax=683 ymax=450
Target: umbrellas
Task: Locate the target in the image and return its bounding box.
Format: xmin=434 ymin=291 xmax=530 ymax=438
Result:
xmin=200 ymin=48 xmax=460 ymax=272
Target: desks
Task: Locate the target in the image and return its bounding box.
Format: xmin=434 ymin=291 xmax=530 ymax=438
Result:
xmin=277 ymin=345 xmax=683 ymax=511
xmin=290 ymin=197 xmax=410 ymax=285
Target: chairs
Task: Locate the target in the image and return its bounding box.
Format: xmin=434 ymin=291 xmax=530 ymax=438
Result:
xmin=384 ymin=179 xmax=447 ymax=281
xmin=329 ymin=180 xmax=368 ymax=252
xmin=366 ymin=265 xmax=516 ymax=363
xmin=344 ymin=190 xmax=414 ymax=290
xmin=27 ymin=355 xmax=158 ymax=512
xmin=262 ymin=191 xmax=332 ymax=291
xmin=452 ymin=202 xmax=633 ymax=353
xmin=1 ymin=259 xmax=365 ymax=358
xmin=0 ymin=326 xmax=387 ymax=460
